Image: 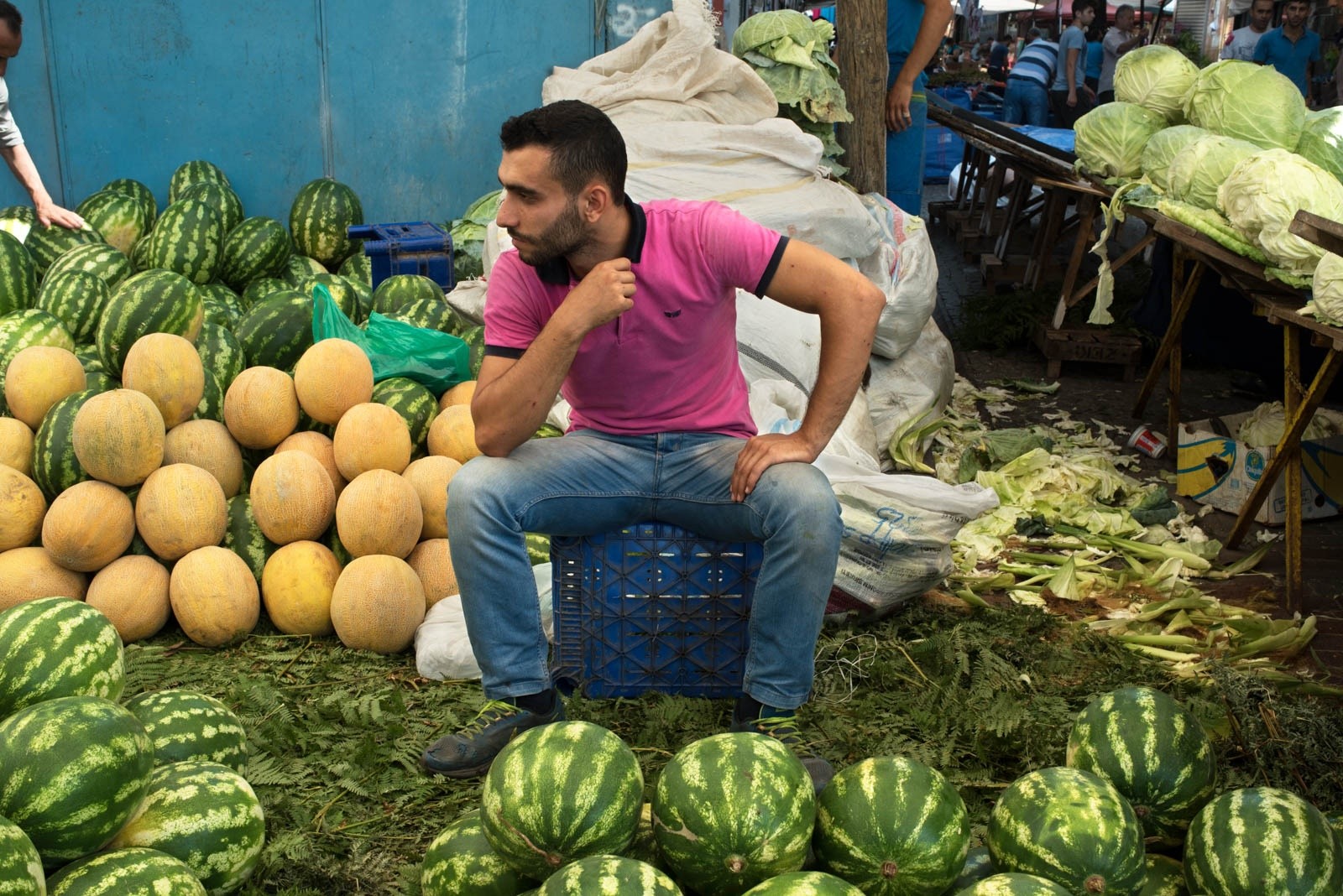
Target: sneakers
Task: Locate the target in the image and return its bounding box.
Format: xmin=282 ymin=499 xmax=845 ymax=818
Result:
xmin=419 ymin=687 xmax=566 ymax=778
xmin=731 ymin=698 xmax=834 ymax=796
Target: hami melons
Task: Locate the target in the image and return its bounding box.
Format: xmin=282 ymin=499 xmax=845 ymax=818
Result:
xmin=0 ymin=334 xmax=485 ymax=655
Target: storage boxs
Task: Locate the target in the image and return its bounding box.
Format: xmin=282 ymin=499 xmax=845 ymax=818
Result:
xmin=1175 ymin=407 xmax=1343 ymax=525
xmin=347 ymin=221 xmax=456 ymax=294
xmin=550 ymin=523 xmax=764 ymax=701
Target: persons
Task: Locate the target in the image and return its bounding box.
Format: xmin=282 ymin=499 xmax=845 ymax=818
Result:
xmin=937 ymin=0 xmax=1342 ymax=133
xmin=0 ymin=1 xmax=90 ymax=230
xmin=423 ymin=99 xmax=885 ymax=795
xmin=823 ymin=1 xmax=955 ymax=218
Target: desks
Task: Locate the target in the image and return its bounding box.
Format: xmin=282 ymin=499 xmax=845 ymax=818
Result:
xmin=944 ymin=106 xmax=1343 ymax=619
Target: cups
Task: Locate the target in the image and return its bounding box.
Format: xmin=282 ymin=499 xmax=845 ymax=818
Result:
xmin=1127 ymin=425 xmax=1166 ymax=459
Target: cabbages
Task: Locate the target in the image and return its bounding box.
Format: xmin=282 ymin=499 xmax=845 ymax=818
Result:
xmin=930 ymin=379 xmax=1225 ymax=572
xmin=1240 ymin=401 xmax=1334 ymax=446
xmin=1071 ymin=45 xmax=1343 ymax=331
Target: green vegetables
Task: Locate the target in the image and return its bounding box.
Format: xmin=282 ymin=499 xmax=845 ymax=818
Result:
xmin=732 ymin=10 xmax=855 ymax=183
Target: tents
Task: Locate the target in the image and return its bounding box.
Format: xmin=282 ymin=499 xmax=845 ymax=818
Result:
xmin=1019 ymin=2 xmax=1150 ymax=42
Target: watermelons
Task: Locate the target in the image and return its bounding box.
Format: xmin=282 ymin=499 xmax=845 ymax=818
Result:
xmin=0 ymin=595 xmax=266 ymax=896
xmin=421 ymin=685 xmax=1343 ymax=896
xmin=0 ymin=161 xmax=564 ymax=583
